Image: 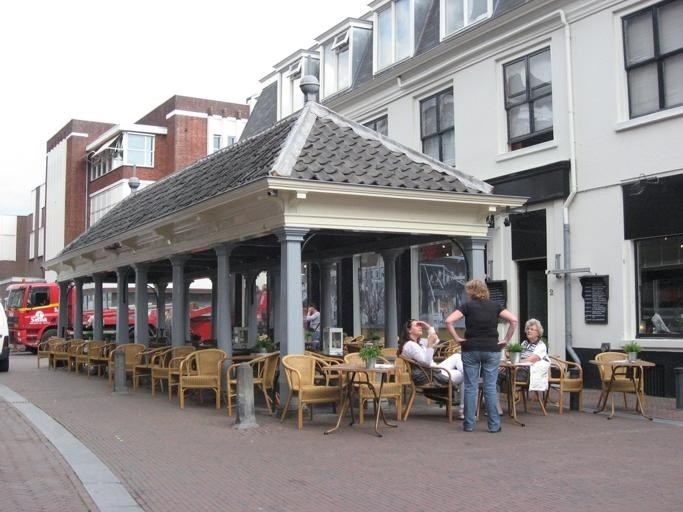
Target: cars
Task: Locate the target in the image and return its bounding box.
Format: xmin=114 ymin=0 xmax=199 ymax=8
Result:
xmin=0 ymin=303 xmax=9 ymax=371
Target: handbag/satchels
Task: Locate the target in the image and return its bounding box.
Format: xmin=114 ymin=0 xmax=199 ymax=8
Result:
xmin=307 ymin=328 xmax=316 ymax=337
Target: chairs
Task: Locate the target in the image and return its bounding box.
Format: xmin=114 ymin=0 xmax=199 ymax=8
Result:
xmin=38 ymin=337 xmax=280 ymax=418
xmin=342 ymin=337 xmax=383 ymax=353
xmin=344 ymin=353 xmax=401 ymax=422
xmin=595 ymin=352 xmax=641 ymax=411
xmin=282 ymin=353 xmax=342 ymax=434
xmin=434 ymin=334 xmax=546 ymax=428
xmin=542 ymin=356 xmax=583 ymax=414
xmin=399 ymin=353 xmax=453 ymax=423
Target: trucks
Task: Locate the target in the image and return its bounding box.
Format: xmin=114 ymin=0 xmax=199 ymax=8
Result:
xmin=3 ymin=282 xmax=213 ymax=355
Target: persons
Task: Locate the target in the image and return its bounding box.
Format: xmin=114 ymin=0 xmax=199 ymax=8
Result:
xmin=444 ymin=280 xmax=519 ymax=433
xmin=398 ymin=319 xmax=465 ymax=420
xmin=307 ymin=302 xmax=321 ymax=350
xmin=483 ymin=318 xmax=551 ymax=416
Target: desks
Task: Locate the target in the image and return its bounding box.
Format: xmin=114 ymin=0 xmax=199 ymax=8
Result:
xmin=588 ymin=360 xmax=656 ymax=420
xmin=321 ymin=363 xmax=399 ymax=437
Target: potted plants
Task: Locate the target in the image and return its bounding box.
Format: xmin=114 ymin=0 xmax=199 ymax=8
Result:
xmin=623 ymin=340 xmax=641 ymax=361
xmin=360 ymin=345 xmax=383 ymax=367
